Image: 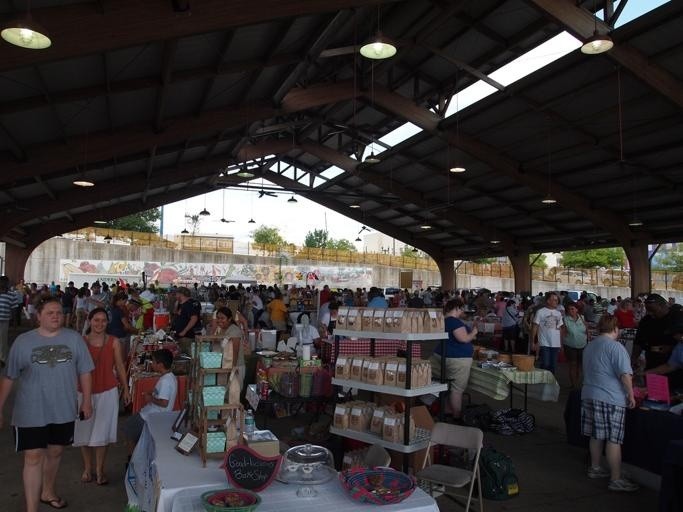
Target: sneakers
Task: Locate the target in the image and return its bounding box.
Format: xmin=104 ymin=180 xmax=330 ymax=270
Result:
xmin=606 ymin=475 xmax=636 ymax=491
xmin=587 ymin=465 xmax=611 ymax=480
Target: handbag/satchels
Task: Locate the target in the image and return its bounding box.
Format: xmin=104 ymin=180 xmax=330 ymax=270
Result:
xmin=438 ymin=444 xmax=520 ymax=502
xmin=490 ymin=407 xmax=539 ymax=436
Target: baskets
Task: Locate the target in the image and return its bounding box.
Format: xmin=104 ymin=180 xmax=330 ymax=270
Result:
xmin=200 ymin=488 xmax=262 ymax=512
xmin=496 ymin=354 xmax=535 ymax=371
xmin=339 ymin=466 xmax=417 ymax=506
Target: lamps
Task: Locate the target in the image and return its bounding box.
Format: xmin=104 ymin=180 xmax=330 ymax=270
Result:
xmin=178 ymin=187 xmax=362 ymax=236
xmin=362 ymin=64 xmax=381 ymax=164
xmin=358 ymin=9 xmax=400 ymax=62
xmin=578 ymin=0 xmax=615 ymax=55
xmin=0 ymin=0 xmax=56 ymax=54
xmin=539 ymin=134 xmax=559 ymax=206
xmin=70 ymin=173 xmax=96 ymax=189
xmin=446 ymin=58 xmax=467 ymax=174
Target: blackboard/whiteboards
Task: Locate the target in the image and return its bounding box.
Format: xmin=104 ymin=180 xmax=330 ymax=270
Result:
xmin=219 ymin=443 xmax=281 ymax=493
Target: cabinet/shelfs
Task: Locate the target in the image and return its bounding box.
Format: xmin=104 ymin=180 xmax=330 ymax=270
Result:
xmin=330 ymin=328 xmax=451 ymax=499
xmin=189 ymin=337 xmax=246 ymax=467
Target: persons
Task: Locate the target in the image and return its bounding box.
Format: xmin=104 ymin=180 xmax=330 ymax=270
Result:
xmin=3 ymin=276 xmax=342 ymax=439
xmin=579 ymin=313 xmax=640 ymax=490
xmin=0 ymin=294 xmax=96 ymax=511
xmin=345 ymin=285 xmax=683 ymax=421
xmin=72 ymin=307 xmax=131 ymax=484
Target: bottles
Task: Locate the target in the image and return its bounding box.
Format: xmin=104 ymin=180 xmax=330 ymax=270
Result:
xmin=244 ymin=410 xmax=255 ymax=434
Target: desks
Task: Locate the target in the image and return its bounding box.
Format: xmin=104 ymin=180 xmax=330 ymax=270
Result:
xmin=139 ymin=412 xmax=285 ymax=512
xmin=176 ymin=466 xmax=434 ymax=511
xmin=129 ymin=334 xmax=191 ymax=418
xmin=564 ymin=387 xmax=682 ymax=480
xmin=469 ymin=361 xmax=560 ymax=434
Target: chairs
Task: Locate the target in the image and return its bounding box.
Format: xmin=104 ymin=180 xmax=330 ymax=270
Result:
xmin=406 ymin=421 xmax=487 ymax=511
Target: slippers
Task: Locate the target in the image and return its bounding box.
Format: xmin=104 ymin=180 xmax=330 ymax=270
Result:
xmin=39 ymin=496 xmax=67 ymax=508
